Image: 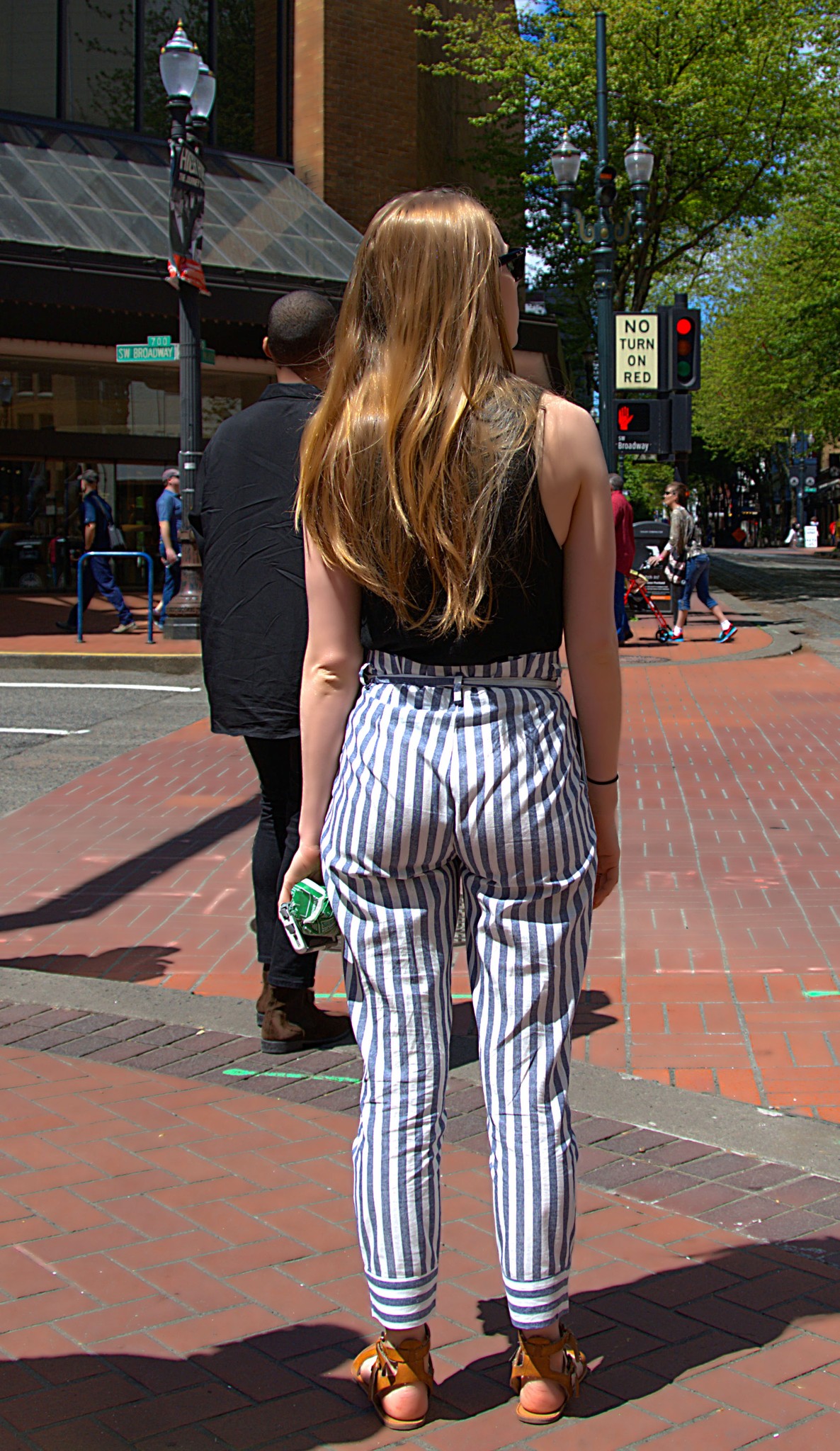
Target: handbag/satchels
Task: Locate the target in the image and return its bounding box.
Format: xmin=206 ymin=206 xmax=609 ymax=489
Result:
xmin=108 ymin=524 xmax=124 ymax=549
xmin=664 ymin=550 xmax=686 ymax=584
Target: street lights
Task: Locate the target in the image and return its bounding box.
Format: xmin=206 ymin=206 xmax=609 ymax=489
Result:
xmin=551 ymin=10 xmax=656 ymax=471
xmin=159 ymin=21 xmax=218 ymax=640
xmin=791 ymin=425 xmax=815 ymax=548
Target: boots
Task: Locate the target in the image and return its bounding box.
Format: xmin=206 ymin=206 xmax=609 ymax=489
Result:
xmin=261 ymin=987 xmax=356 ymax=1053
xmin=257 ymin=970 xmax=315 ymax=1025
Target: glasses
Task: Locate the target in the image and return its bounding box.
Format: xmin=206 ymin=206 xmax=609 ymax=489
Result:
xmin=492 ymin=247 xmax=526 ymax=282
xmin=663 ymin=491 xmax=676 ymax=495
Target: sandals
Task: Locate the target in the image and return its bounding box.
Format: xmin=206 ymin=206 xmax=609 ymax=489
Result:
xmin=510 ymin=1324 xmax=587 ymax=1423
xmin=353 ymin=1323 xmax=434 ymax=1430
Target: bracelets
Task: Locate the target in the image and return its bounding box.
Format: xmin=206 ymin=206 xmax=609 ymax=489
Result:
xmin=659 ymin=555 xmax=664 ymax=559
xmin=586 ymin=775 xmax=618 ymax=785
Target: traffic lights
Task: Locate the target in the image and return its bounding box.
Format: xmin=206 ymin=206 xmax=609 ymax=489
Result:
xmin=610 ymin=397 xmax=653 ymax=454
xmin=668 ymin=306 xmax=701 ymax=391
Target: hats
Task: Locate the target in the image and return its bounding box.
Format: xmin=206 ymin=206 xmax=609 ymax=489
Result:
xmin=162 ymin=468 xmax=179 ymax=482
xmin=77 ymin=470 xmax=99 ymax=482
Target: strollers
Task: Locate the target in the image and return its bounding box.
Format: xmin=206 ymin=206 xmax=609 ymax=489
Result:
xmin=623 ymin=561 xmax=672 ymax=643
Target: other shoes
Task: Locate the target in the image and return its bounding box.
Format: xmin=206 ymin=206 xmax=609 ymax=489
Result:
xmin=54 ymin=622 xmax=76 ymax=632
xmin=152 ymin=609 xmax=160 ymax=626
xmin=112 ymin=622 xmax=137 ymax=633
xmin=618 ymin=630 xmax=633 ymax=646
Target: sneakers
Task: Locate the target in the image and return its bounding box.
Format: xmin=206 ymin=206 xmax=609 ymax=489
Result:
xmin=660 ymin=631 xmax=684 ymax=642
xmin=716 ymin=623 xmax=738 ymax=643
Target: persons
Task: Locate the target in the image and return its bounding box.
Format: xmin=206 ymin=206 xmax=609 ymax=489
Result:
xmin=153 ymin=468 xmax=182 ymax=629
xmin=128 ymin=484 xmax=144 ymax=580
xmin=54 ymin=469 xmax=137 ymax=633
xmin=188 ymin=291 xmax=337 ymax=1058
xmin=277 ymin=187 xmax=622 ymax=1426
xmin=791 ymin=517 xmax=800 ymax=548
xmin=829 ymin=521 xmax=836 ymax=545
xmin=809 ymin=516 xmax=819 ymax=536
xmin=646 ymin=482 xmax=738 ymax=643
xmin=48 ymin=527 xmax=76 ymax=594
xmin=609 ymin=473 xmax=636 ymax=645
